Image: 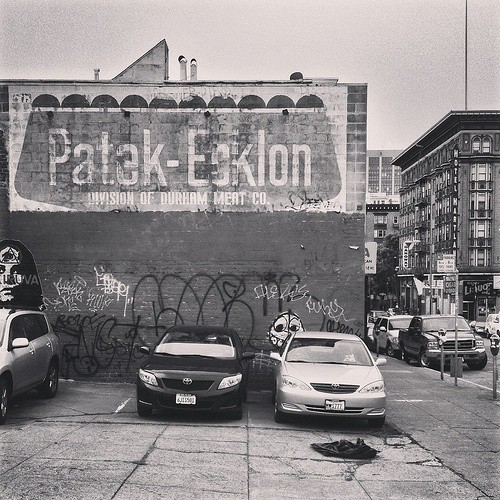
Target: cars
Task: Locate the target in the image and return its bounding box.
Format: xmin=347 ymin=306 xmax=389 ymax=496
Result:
xmin=136 ymin=324 xmax=255 ymax=421
xmin=469 ymin=314 xmax=500 ymax=339
xmin=269 ymin=329 xmax=389 ymax=428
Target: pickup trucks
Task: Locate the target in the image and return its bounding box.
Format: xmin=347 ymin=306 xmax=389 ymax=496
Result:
xmin=399 ymin=314 xmax=488 ymax=370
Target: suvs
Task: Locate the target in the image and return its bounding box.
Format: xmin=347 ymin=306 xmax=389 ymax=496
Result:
xmin=372 ymin=315 xmax=415 ymax=357
xmin=368 ymin=309 xmax=388 ymax=323
xmin=0 ymin=303 xmax=62 ymax=427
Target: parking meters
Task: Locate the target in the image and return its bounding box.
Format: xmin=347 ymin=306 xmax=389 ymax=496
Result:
xmin=437 ymin=328 xmax=447 ymax=381
xmin=374 ymin=324 xmax=381 ymax=358
xmin=489 ymin=333 xmax=500 ymax=400
